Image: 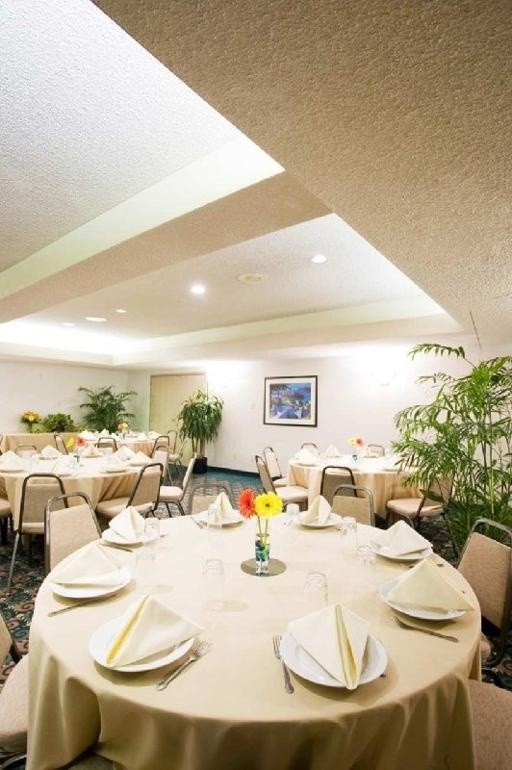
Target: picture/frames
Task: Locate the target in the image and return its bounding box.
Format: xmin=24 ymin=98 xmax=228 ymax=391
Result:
xmin=264 ymin=375 xmax=317 ymax=427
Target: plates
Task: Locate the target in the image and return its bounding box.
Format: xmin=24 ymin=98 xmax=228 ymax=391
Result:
xmin=88 ymin=614 xmax=195 ymax=674
xmin=279 ymin=630 xmax=389 ymax=688
xmin=198 ymin=511 xmax=243 ymax=525
xmin=46 ymin=559 xmax=132 ymax=599
xmin=100 ymin=524 xmax=165 ymax=544
xmin=78 ymin=429 xmax=161 ymax=442
xmin=295 ymin=512 xmax=340 ymax=528
xmin=378 ymin=579 xmax=468 ymax=620
xmin=0 ymin=444 xmax=154 ymax=477
xmin=369 ymin=536 xmax=434 ymax=562
xmin=294 ymin=444 xmax=404 ymax=472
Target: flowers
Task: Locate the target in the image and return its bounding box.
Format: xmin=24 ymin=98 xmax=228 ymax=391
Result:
xmin=117 ymin=422 xmax=129 ymax=430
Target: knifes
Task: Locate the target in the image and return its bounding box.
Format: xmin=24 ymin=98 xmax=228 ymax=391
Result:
xmin=190 ymin=517 xmax=203 ymax=531
xmin=101 ymin=542 xmax=131 ymax=552
xmin=47 ymin=593 xmax=120 ymax=616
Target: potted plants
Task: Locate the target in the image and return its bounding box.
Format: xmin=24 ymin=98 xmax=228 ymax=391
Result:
xmin=173 ymin=389 xmax=223 ymax=474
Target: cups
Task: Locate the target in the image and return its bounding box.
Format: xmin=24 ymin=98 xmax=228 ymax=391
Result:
xmin=287 ymin=504 xmax=299 ymax=529
xmin=142 ymin=517 xmax=161 ymax=547
xmin=206 ymin=505 xmax=225 ymax=533
xmin=336 ymin=517 xmax=359 ymax=555
xmin=200 ymin=559 xmax=226 ymax=611
xmin=304 ymin=572 xmax=328 ymax=611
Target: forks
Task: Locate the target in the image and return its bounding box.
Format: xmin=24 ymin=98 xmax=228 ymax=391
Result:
xmin=272 ymin=635 xmax=302 ymax=692
xmin=393 ymin=615 xmax=458 ymax=643
xmin=154 ymin=641 xmax=211 ymax=690
